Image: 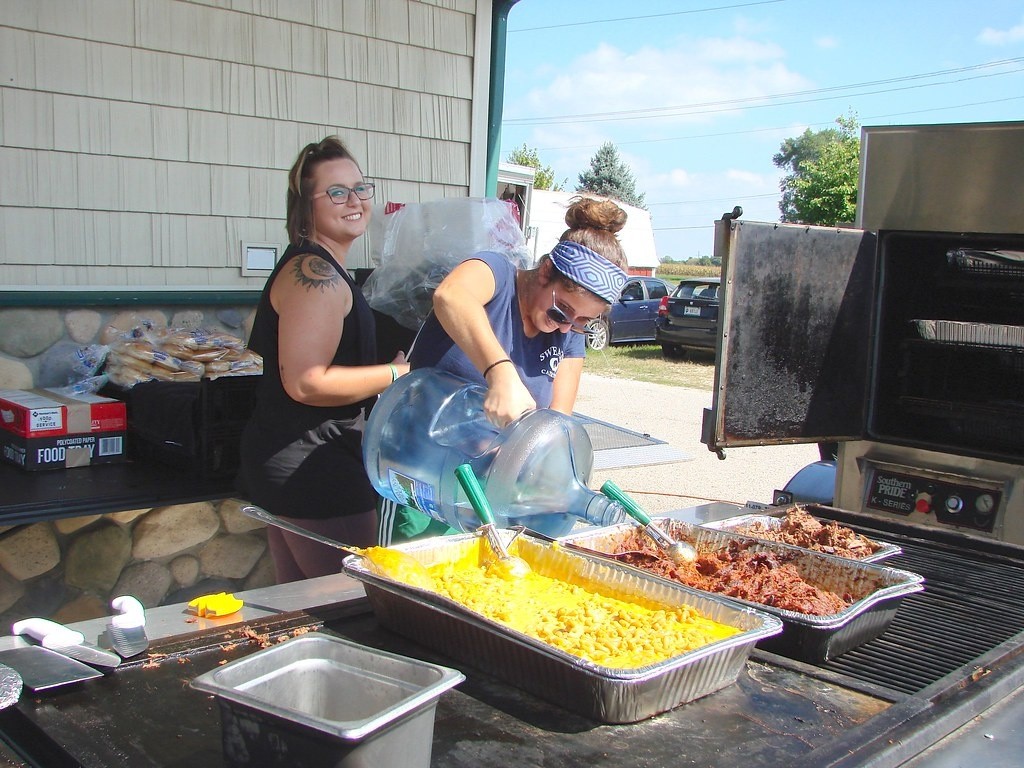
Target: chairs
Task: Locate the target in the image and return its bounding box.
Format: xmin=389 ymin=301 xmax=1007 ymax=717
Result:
xmin=700 ymin=289 xmax=715 ymax=297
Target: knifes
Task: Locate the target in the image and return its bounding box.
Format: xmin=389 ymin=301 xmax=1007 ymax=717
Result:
xmin=105 ymin=595 xmax=149 ymax=658
xmin=12 ymin=617 xmax=121 ymax=667
xmin=0 ymin=645 xmax=104 ymax=691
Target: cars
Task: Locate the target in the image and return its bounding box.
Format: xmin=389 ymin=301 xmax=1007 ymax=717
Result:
xmin=584 ymin=276 xmax=678 ymax=353
xmin=656 ymin=279 xmax=722 ymax=358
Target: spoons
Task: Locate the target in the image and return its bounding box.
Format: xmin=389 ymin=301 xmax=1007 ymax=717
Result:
xmin=239 ymin=504 xmax=435 ymax=595
xmin=506 ymin=525 xmax=661 ymax=567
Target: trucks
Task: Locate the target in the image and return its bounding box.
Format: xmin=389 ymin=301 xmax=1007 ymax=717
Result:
xmin=703 ymin=119 xmax=1024 ymax=549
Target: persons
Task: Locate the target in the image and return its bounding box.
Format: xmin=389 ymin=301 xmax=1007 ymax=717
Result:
xmin=376 ymin=197 xmax=631 ymax=548
xmin=239 ymin=135 xmax=411 ymax=585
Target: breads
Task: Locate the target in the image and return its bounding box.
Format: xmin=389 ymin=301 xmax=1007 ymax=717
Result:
xmin=100 ymin=325 xmax=264 ymax=387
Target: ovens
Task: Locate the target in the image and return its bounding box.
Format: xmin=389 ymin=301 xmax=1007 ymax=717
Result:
xmin=701 ymin=206 xmax=1023 ymax=546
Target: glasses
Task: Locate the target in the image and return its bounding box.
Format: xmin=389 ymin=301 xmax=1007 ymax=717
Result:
xmin=545 ymin=283 xmax=603 ymax=337
xmin=311 ymin=182 xmax=378 ymax=205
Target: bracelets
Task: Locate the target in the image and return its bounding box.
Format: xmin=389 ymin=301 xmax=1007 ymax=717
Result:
xmin=388 ymin=363 xmax=399 ymax=383
xmin=483 ymin=359 xmax=513 ymax=378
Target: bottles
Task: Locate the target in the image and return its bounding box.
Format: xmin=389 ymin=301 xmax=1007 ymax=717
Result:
xmin=362 ymin=365 xmax=626 ymax=538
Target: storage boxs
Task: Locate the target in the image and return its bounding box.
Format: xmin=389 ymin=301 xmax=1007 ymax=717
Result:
xmin=0 ymin=386 xmax=128 ymax=472
xmin=341 ymin=513 xmax=924 ymax=725
